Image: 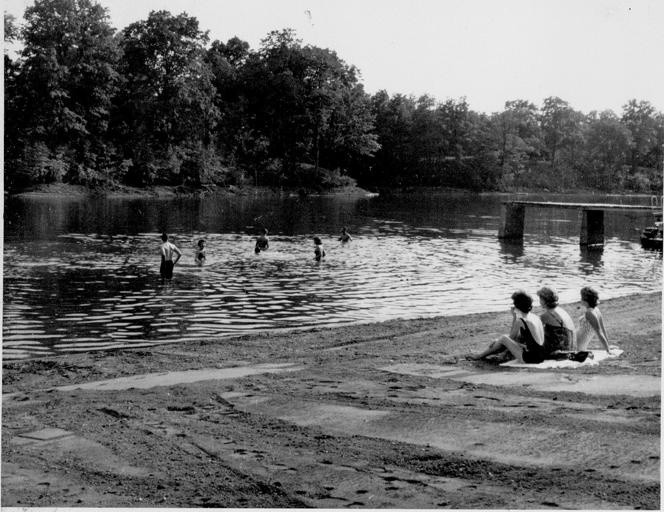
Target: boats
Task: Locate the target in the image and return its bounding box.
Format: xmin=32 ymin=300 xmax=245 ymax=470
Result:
xmin=640 ymin=226 xmax=663 ymax=251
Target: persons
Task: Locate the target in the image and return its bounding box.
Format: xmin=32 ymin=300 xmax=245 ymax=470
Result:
xmin=160 ymin=233 xmax=182 ymax=273
xmin=195 ymin=240 xmax=208 ymax=265
xmin=313 ymin=236 xmax=326 ymax=262
xmin=256 ymin=228 xmax=269 ymax=253
xmin=337 ymin=225 xmax=353 ymax=243
xmin=465 ymin=286 xmax=617 ymax=364
